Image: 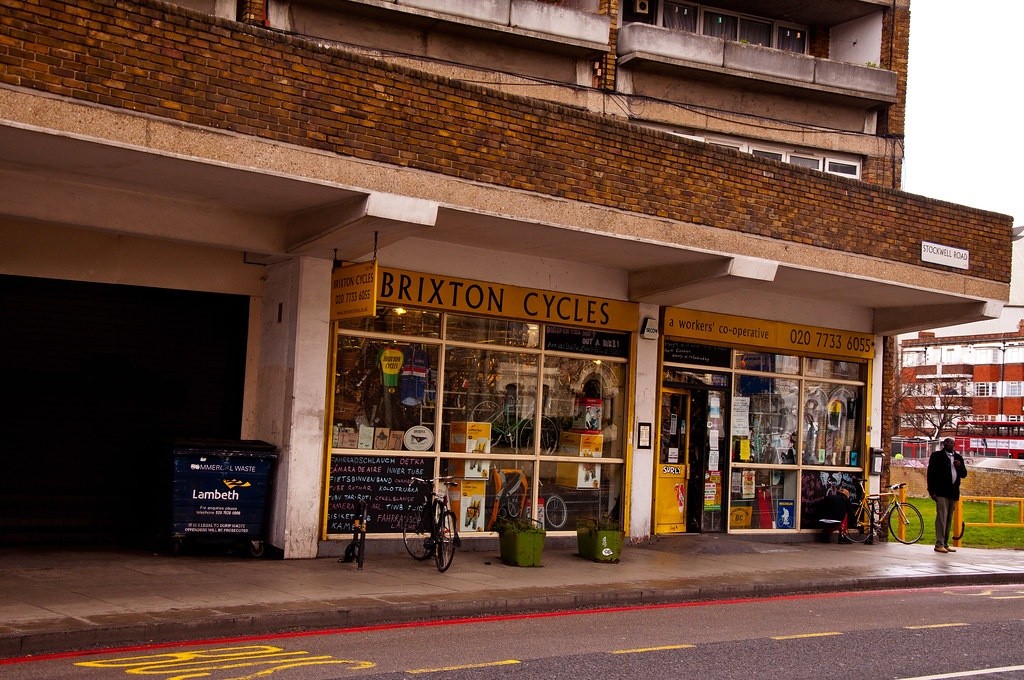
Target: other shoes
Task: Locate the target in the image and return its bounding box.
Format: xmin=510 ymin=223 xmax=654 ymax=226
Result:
xmin=825 ymin=533 xmax=838 ymax=543
xmin=838 ymin=534 xmax=853 ymax=544
xmin=945 ymin=546 xmax=956 ymax=552
xmin=934 ymin=545 xmax=948 ymax=553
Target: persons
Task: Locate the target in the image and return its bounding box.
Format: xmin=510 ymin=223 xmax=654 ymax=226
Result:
xmin=818 ymin=489 xmax=858 ymax=544
xmin=927 ymin=438 xmax=967 ymax=552
xmin=895 ymin=451 xmax=903 ymax=458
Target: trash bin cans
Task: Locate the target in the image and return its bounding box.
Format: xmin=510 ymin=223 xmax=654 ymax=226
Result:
xmin=156 ymin=437 xmax=281 ymax=560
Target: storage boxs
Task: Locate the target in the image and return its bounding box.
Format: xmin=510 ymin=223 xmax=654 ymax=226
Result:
xmin=449 ymin=421 xmax=489 ymax=480
xmin=446 ymin=478 xmax=486 ymax=532
xmin=558 ymin=432 xmax=603 ymax=489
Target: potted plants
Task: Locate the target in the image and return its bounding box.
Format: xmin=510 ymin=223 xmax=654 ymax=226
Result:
xmin=491 ymin=514 xmax=546 ymax=567
xmin=577 ymin=517 xmax=625 ymax=563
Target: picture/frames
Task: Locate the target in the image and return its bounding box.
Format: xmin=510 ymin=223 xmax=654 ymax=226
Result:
xmin=637 ymin=423 xmax=652 ymax=449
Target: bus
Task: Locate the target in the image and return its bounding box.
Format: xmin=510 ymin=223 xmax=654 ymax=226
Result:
xmin=955 ymin=422 xmax=1024 ymax=459
xmin=892 ymin=436 xmax=932 ymax=459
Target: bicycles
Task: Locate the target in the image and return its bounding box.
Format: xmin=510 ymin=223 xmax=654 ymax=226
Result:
xmin=497 ymin=478 xmax=569 ymax=529
xmin=841 ymin=478 xmax=924 ymax=545
xmin=758 ymin=426 xmax=789 ymax=485
xmin=403 ymin=475 xmax=459 ymax=573
xmin=801 ymin=371 xmax=856 ymax=416
xmin=467 ymin=383 xmax=559 ymax=455
